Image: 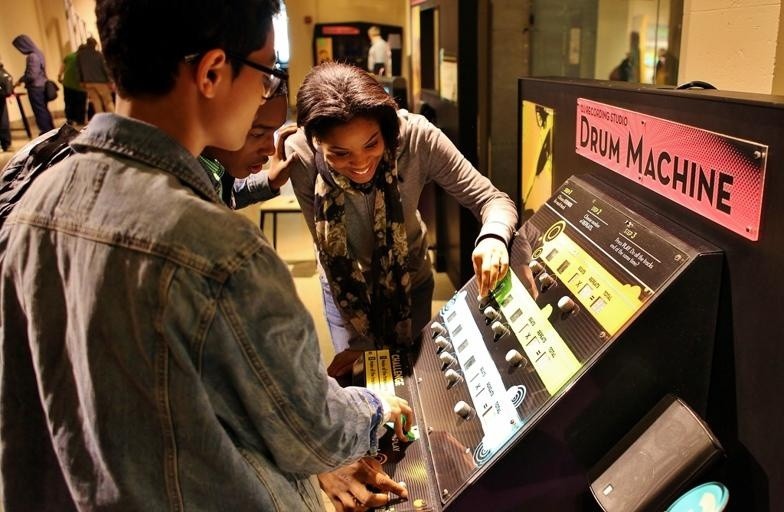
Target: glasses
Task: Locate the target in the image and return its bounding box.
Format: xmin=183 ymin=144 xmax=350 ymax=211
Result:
xmin=184 ymin=52 xmax=288 ymax=101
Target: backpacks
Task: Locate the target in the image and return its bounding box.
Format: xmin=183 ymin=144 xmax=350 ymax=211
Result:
xmin=0 ymin=67 xmax=13 ymax=98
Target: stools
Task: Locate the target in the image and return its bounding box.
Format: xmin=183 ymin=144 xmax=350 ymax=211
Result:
xmin=259 ymin=194 xmax=316 ymax=251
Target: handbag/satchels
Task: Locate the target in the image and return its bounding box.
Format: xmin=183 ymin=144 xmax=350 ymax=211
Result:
xmin=44 ymin=80 xmax=59 ymax=102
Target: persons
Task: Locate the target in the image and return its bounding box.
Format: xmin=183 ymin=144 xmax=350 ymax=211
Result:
xmin=504 ymin=218 xmax=542 ymax=300
xmin=283 ymin=60 xmax=518 ymax=360
xmin=0 ymin=64 xmax=297 ymax=213
xmin=650 ymin=47 xmax=666 ymax=84
xmin=0 ymin=33 xmax=115 ymax=153
xmin=366 ymin=25 xmax=391 ymax=74
xmin=606 ymin=56 xmax=628 ymax=81
xmin=0 ymin=1 xmax=416 ymax=510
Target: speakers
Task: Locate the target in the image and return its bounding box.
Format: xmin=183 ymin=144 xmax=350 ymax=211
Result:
xmin=585 ymin=392 xmax=726 ymax=511
